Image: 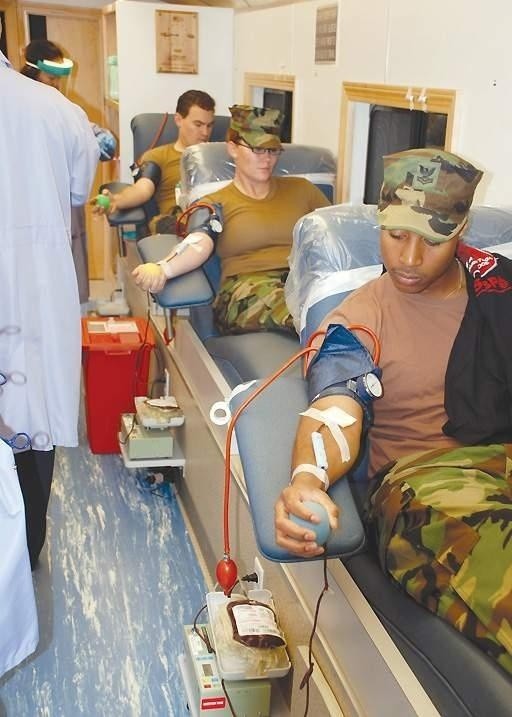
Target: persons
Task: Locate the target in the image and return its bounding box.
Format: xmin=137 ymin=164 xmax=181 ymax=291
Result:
xmin=1 ymin=36 xmax=98 ymax=568
xmin=90 ymin=89 xmax=216 ymax=236
xmin=132 ymin=104 xmax=333 ymax=334
xmin=21 ymin=37 xmax=118 ymax=302
xmin=273 ymin=148 xmax=512 ymax=683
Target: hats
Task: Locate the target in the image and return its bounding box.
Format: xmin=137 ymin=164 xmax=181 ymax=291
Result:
xmin=227 ymin=104 xmax=286 ymax=150
xmin=378 ymin=148 xmax=484 ymax=242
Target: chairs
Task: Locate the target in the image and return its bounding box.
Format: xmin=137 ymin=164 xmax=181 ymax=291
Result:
xmin=99 ymin=112 xmax=511 ymax=715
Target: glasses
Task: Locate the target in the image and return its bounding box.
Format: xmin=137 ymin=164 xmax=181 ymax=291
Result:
xmin=236 ymin=142 xmax=283 ymax=155
